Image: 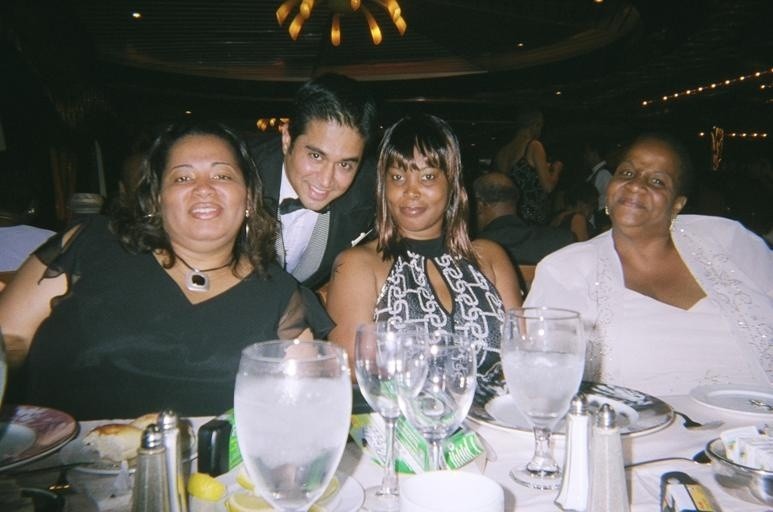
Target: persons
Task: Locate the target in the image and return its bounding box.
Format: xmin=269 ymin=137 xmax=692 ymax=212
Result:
xmin=245 ymin=69 xmax=378 ymax=290
xmin=520 ymin=128 xmax=773 ymax=397
xmin=325 ymin=111 xmax=528 ymax=413
xmin=0 ymin=112 xmax=336 ymax=421
xmin=671 ymin=131 xmax=734 ymax=213
xmin=473 ymin=112 xmax=612 ymax=294
xmin=116 ymin=150 xmax=150 ymax=208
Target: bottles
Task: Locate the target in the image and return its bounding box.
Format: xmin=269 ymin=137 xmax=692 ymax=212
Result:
xmin=589 ymin=403 xmax=631 ymax=511
xmin=132 ymin=423 xmax=168 ymax=511
xmin=156 ymin=407 xmax=190 ymax=511
xmin=553 ymin=393 xmax=595 ymax=511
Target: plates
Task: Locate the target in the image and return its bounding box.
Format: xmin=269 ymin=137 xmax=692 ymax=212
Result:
xmin=59 ymin=428 xmax=202 ymax=475
xmin=0 ymin=403 xmax=80 ymax=468
xmin=467 ymin=379 xmax=676 ymax=443
xmin=226 ymin=469 xmax=365 ymax=512
xmin=689 ymin=383 xmax=773 ymax=416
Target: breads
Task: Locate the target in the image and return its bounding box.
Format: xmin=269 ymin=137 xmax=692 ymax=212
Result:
xmin=82 ymin=412 xmax=159 ymax=463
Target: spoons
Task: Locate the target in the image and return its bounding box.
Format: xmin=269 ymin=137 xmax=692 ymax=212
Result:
xmin=624 ymin=448 xmax=711 ymax=471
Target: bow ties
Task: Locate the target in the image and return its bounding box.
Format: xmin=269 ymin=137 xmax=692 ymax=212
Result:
xmin=280 ymin=198 xmax=332 ymax=215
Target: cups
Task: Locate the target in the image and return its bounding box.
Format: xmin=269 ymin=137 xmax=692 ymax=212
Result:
xmin=232 ymin=339 xmax=353 ymax=511
xmin=403 ymin=466 xmax=509 ymax=512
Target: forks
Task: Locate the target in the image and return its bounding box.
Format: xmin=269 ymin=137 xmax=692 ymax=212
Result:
xmin=671 ymin=409 xmax=723 ymax=434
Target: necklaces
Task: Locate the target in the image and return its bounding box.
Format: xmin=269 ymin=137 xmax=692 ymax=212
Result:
xmin=172 ymin=251 xmax=237 ymax=292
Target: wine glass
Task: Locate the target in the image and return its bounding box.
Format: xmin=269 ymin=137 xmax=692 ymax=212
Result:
xmin=393 ymin=325 xmax=479 ymax=472
xmin=353 ymin=321 xmax=425 ymax=512
xmin=501 ymin=306 xmax=587 ymax=491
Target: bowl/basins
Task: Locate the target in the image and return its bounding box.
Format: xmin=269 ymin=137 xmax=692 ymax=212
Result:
xmin=704 ymin=438 xmax=773 ymax=507
